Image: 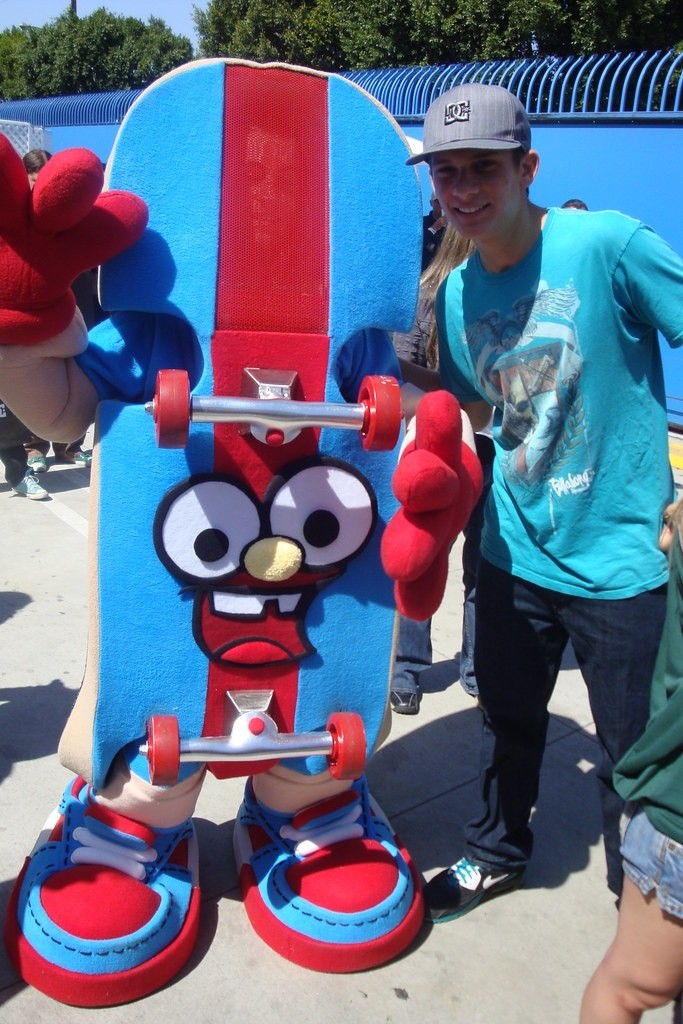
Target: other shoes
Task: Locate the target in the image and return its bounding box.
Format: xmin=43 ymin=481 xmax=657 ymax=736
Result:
xmin=390 ymin=692 xmax=418 ymax=715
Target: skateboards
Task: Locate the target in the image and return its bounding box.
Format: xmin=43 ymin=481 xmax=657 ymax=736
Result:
xmin=79 ymin=54 xmax=444 ymax=802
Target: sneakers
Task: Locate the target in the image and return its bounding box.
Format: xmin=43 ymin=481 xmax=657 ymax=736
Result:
xmin=55 ymin=449 xmax=92 ymax=466
xmin=12 ymin=475 xmax=49 ymax=500
xmin=27 ymin=456 xmax=46 ymax=471
xmin=423 ymin=858 xmax=525 ymax=924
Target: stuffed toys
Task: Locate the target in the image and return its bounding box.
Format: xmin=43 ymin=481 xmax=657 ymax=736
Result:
xmin=0 ymin=58 xmax=488 ymax=1008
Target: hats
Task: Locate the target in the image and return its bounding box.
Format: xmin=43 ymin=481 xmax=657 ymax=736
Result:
xmin=405 ymin=83 xmax=531 ymax=166
xmin=431 ymin=192 xmax=438 ymax=201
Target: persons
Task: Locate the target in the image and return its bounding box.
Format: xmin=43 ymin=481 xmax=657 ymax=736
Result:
xmin=388 ymin=190 xmax=589 ymax=714
xmin=404 ymin=83 xmax=683 ymax=922
xmin=577 ymin=497 xmax=683 ymax=1024
xmin=0 ymin=149 xmax=102 ymax=500
